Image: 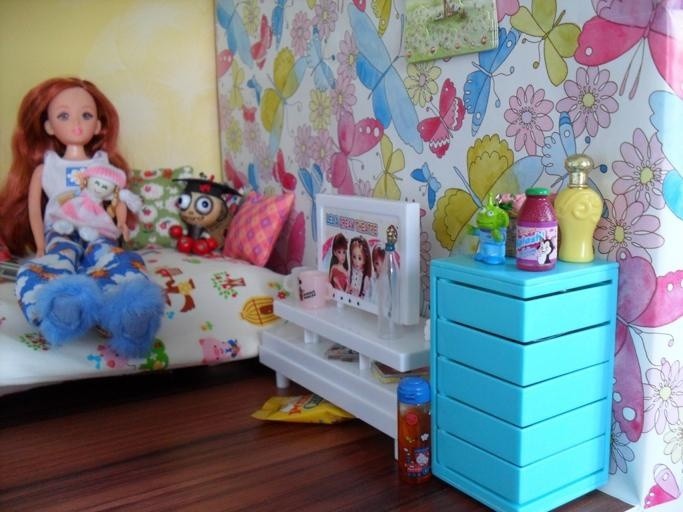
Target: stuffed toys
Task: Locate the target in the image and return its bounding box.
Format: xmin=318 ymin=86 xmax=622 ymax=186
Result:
xmin=466 ymin=191 xmax=510 ymax=265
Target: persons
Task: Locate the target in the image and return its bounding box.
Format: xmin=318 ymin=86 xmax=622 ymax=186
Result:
xmin=172 ymin=174 xmax=244 ymax=256
xmin=0 ymin=77 xmax=167 ymax=358
xmin=49 ymin=164 xmax=128 ymax=242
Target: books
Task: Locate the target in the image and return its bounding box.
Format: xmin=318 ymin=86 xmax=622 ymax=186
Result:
xmin=371 ymin=360 xmax=429 ymax=384
xmin=326 ymin=343 xmax=359 ymax=363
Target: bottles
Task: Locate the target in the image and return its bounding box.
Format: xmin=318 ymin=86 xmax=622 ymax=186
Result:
xmin=378 ymin=243 xmax=400 ymax=337
xmin=397 ymin=376 xmax=433 ymax=485
xmin=517 ymin=154 xmax=605 ymax=270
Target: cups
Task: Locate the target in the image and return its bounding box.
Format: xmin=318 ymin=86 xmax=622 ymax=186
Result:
xmin=284 ymin=266 xmax=333 ymax=307
